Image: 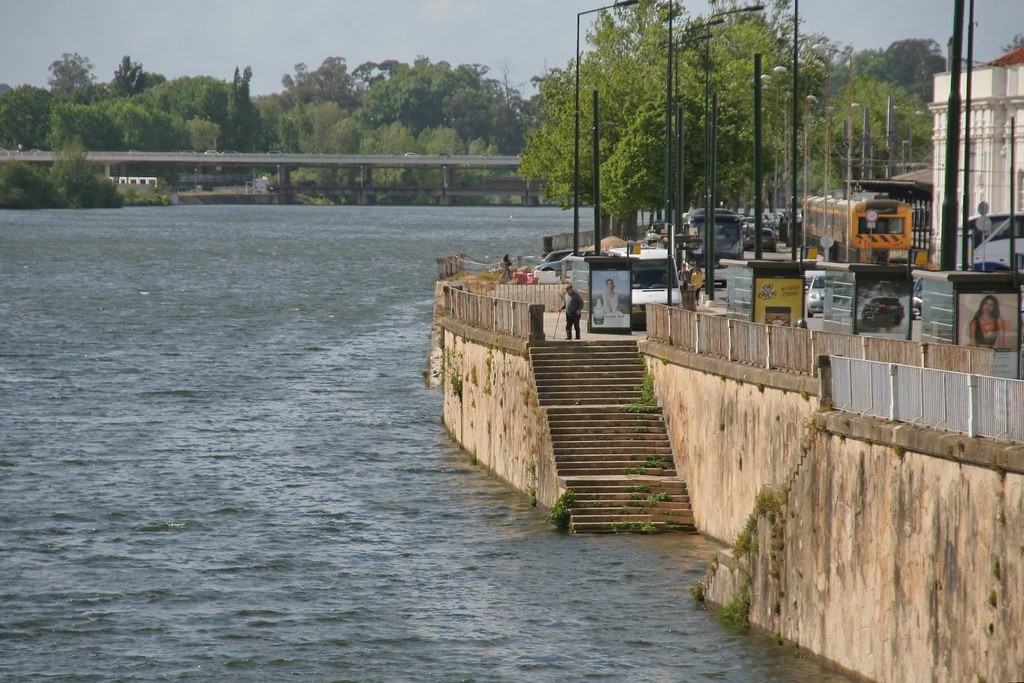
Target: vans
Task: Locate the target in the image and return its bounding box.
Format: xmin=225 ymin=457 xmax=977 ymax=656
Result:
xmin=608 ymin=245 xmax=684 ymax=331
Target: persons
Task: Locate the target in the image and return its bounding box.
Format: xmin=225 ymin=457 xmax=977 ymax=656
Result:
xmin=677 ymin=261 xmax=703 ymax=306
xmin=602 ymin=279 xmax=623 ymax=314
xmin=500 ymin=254 xmax=513 ymax=280
xmin=780 ymin=213 xmax=788 ymax=224
xmin=966 ymin=295 xmax=1005 ymax=349
xmin=558 ymin=285 xmax=585 ymax=340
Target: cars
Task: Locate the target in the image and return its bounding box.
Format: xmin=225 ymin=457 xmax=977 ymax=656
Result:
xmin=913 ymin=277 xmax=953 ymax=317
xmin=862 ymin=297 xmax=905 ymax=324
xmin=910 ymin=295 xmax=924 ymax=321
xmin=805 ymin=270 xmax=825 ymax=291
xmin=534 ymin=206 xmax=804 ymax=291
xmin=804 ymin=272 xmax=845 ymax=319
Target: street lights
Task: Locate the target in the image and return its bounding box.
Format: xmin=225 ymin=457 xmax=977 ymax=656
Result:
xmin=674 ymin=17 xmax=725 ymax=232
xmin=704 ymin=4 xmax=766 ymax=297
xmin=811 ymin=42 xmax=852 ymax=263
xmin=571 ymin=0 xmax=640 ymax=257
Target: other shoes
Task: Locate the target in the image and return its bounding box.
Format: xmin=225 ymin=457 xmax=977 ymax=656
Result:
xmin=697 ymin=303 xmax=699 ymax=306
xmin=574 ymin=338 xmax=580 ymax=340
xmin=565 ymin=337 xmax=572 ymax=340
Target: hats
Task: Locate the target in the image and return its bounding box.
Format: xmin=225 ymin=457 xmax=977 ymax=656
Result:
xmin=565 ymin=284 xmax=572 ymax=290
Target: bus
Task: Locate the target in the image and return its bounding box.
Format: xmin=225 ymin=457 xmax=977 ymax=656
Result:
xmin=967 ymin=212 xmax=1023 ymax=314
xmin=801 ymin=193 xmax=913 ymax=265
